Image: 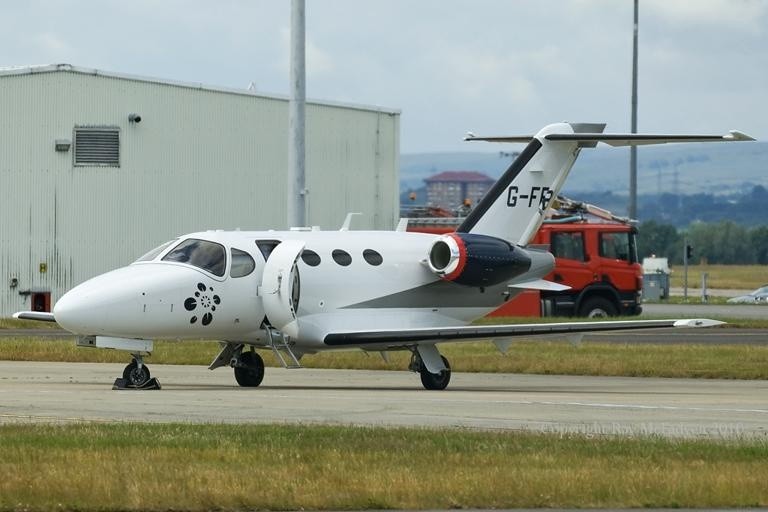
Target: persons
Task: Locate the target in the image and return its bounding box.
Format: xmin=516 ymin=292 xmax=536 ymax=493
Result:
xmin=458 ymin=199 xmax=472 ymax=217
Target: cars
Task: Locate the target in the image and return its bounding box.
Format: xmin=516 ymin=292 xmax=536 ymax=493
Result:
xmin=726 ymin=283 xmax=768 ymax=305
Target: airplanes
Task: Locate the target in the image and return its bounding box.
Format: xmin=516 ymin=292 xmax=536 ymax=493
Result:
xmin=12 ymin=122 xmax=758 ymax=390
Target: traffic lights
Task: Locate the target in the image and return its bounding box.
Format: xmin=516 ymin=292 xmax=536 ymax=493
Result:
xmin=687 ymin=245 xmax=693 ymax=258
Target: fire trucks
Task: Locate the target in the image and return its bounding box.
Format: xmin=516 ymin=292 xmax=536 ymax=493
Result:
xmin=401 ymin=192 xmax=642 ymax=318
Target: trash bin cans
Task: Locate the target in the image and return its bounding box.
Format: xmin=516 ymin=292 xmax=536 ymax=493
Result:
xmin=642 ymin=280 xmax=661 ymax=300
